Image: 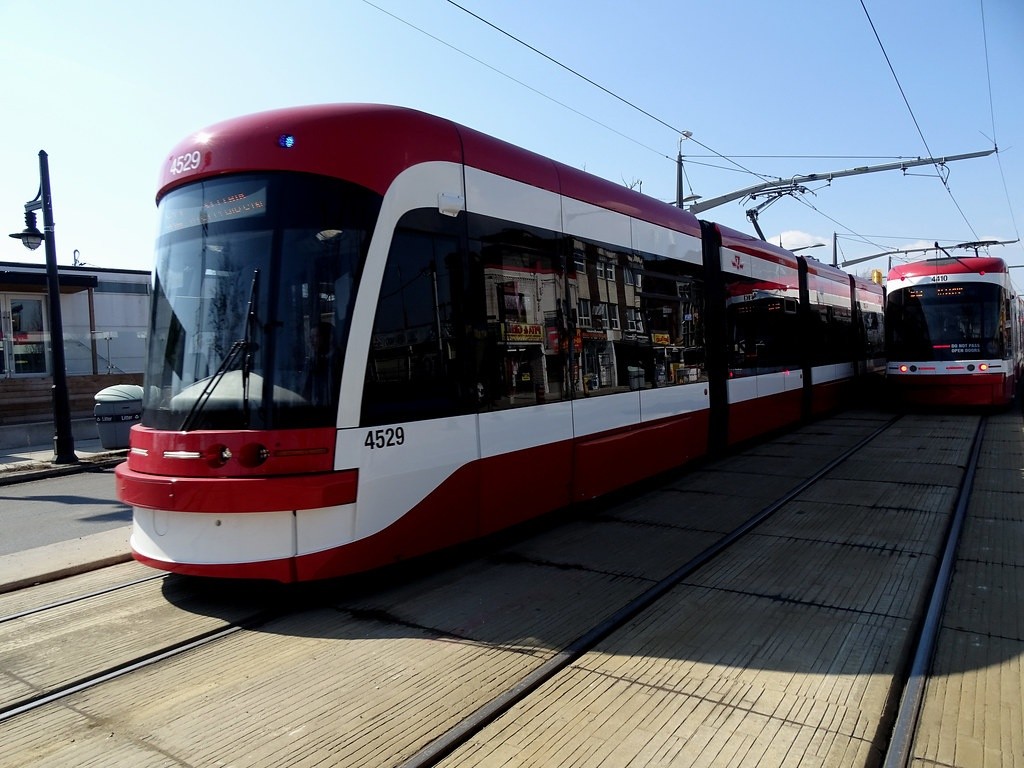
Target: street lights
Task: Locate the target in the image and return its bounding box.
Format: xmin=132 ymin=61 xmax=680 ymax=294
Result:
xmin=676 ymin=131 xmax=693 ymax=210
xmin=9 ymin=150 xmax=78 ymax=463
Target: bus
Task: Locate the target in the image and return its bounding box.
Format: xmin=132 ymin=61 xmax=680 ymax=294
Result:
xmin=725 ymin=291 xmax=799 ymax=376
xmin=886 ymin=246 xmax=1024 ymax=407
xmin=117 ymin=103 xmax=888 ymax=584
